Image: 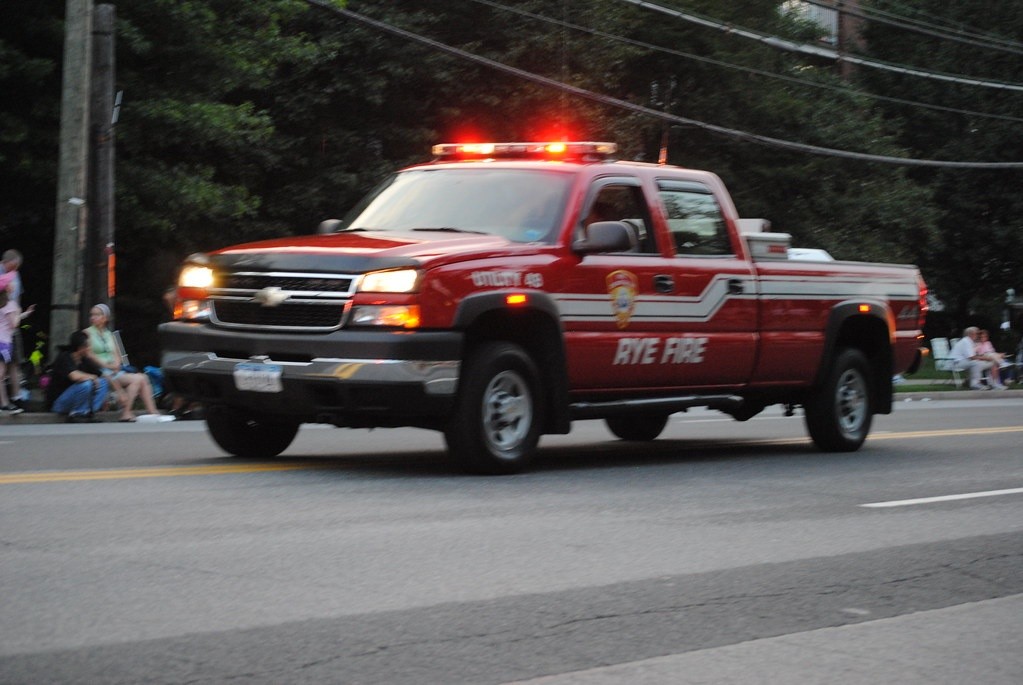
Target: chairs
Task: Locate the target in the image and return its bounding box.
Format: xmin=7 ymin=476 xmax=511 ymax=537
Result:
xmin=645 ymin=230 xmax=703 ymax=254
xmin=930 ymin=338 xmax=965 ymax=390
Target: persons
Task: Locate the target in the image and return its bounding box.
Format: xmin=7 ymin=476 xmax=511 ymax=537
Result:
xmin=82 ymin=304 xmax=160 ymax=423
xmin=946 ymin=327 xmax=1014 ymax=390
xmin=582 ymin=203 xmax=640 ymax=253
xmin=46 ymin=332 xmax=118 ymax=423
xmin=0 ymin=250 xmax=35 ymax=416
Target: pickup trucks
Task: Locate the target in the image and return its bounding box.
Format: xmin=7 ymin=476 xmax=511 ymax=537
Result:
xmin=154 ymin=143 xmax=927 ymax=474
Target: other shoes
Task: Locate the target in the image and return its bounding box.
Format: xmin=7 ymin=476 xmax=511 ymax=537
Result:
xmin=118 ymin=417 xmax=137 ymax=422
xmin=970 ymin=383 xmax=991 ymax=391
xmin=997 ymin=362 xmax=1015 ymax=372
xmin=64 ymin=413 xmax=92 ymax=423
xmin=0 ymin=402 xmax=24 ymax=415
xmin=988 ymin=382 xmax=1010 ymax=390
xmin=86 ymin=412 xmax=103 ymax=423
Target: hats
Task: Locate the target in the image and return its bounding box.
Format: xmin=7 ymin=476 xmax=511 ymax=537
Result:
xmin=94 ymin=303 xmax=111 ymax=317
xmin=0 ymin=270 xmax=17 ymax=291
